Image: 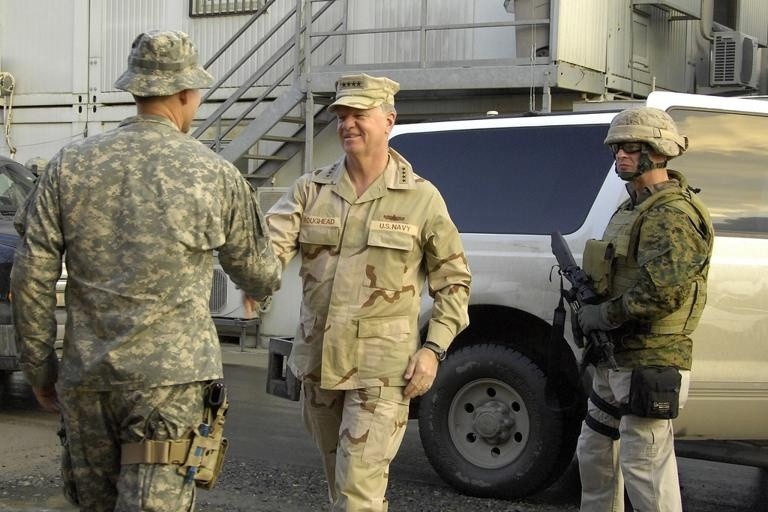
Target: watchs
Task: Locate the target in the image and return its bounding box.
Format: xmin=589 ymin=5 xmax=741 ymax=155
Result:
xmin=421 ymin=343 xmax=447 ymax=364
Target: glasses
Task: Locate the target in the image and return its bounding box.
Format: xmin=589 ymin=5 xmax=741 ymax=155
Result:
xmin=609 ymin=143 xmax=642 ymax=154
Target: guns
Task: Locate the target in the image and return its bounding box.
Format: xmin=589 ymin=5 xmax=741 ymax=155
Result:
xmin=551 ymin=232 xmax=620 ymax=373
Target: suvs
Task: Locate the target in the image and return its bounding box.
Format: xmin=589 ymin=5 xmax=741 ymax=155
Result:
xmin=265 ymin=89 xmax=768 ymax=501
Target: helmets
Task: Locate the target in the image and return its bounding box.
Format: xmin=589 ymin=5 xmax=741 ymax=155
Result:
xmin=604 ymin=105 xmax=689 ymax=159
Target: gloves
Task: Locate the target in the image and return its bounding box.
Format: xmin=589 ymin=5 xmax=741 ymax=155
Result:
xmin=577 ymin=303 xmax=609 ymax=334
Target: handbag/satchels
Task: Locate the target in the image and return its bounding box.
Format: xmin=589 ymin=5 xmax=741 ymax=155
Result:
xmin=628 ymin=366 xmax=683 ymax=419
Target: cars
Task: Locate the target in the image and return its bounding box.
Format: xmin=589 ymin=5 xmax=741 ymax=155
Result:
xmin=0 ymin=150 xmax=67 ymax=375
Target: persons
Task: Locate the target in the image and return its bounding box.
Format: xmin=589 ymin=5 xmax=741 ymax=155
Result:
xmin=238 ymin=70 xmax=475 ymax=512
xmin=557 ymin=103 xmax=716 ymax=512
xmin=10 ymin=28 xmax=284 ymax=512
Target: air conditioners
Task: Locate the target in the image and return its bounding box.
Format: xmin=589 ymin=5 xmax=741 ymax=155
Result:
xmin=711 ymin=30 xmax=760 ymax=90
xmin=207 ymin=266 xmax=259 ymax=319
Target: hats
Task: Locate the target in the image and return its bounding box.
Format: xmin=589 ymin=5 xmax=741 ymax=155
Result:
xmin=326 ymin=72 xmax=401 ymax=113
xmin=113 ymin=29 xmax=216 ymax=97
